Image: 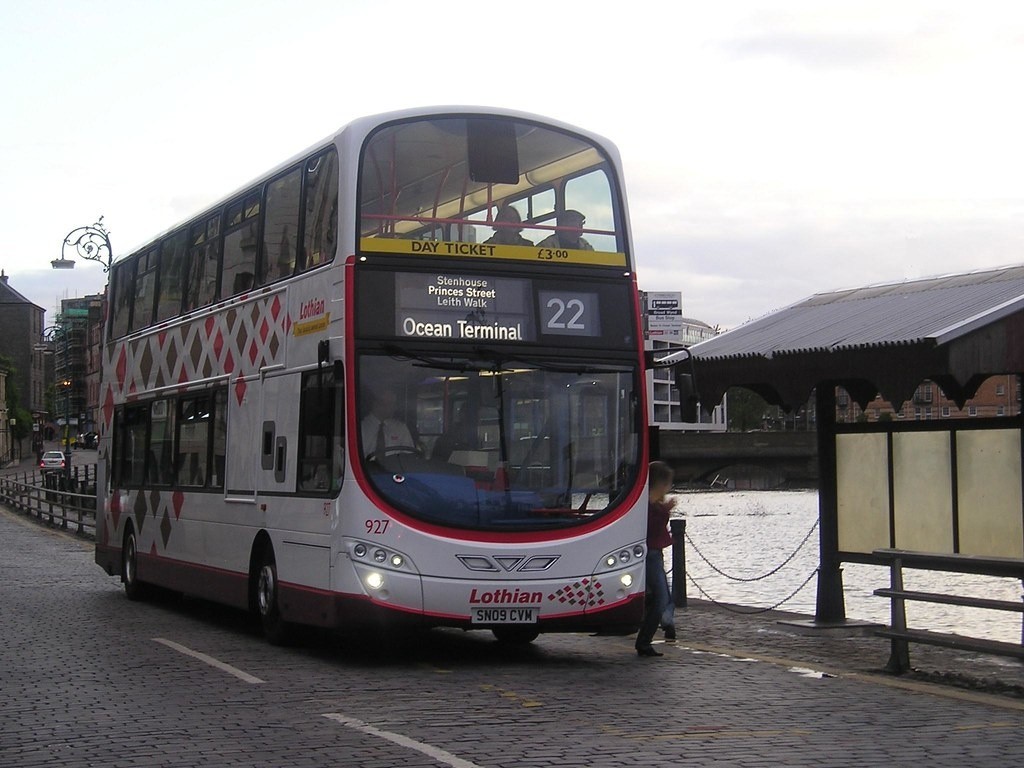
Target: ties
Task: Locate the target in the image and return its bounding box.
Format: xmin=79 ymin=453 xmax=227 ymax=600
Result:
xmin=374 ymin=422 xmax=385 ymax=469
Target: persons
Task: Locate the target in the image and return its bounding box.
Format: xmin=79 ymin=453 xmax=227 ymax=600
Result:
xmin=431 ymin=403 xmax=492 ymax=479
xmin=536 ymin=210 xmax=596 ymax=251
xmin=356 ymin=396 xmax=418 ymax=467
xmin=483 ymin=206 xmax=535 ymax=247
xmin=74 ymin=432 xmax=96 ymax=450
xmin=635 ymin=462 xmax=676 ymax=656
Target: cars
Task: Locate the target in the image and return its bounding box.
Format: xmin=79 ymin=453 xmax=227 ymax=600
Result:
xmin=61 ymin=430 xmax=100 ymax=451
xmin=39 ymin=451 xmax=66 ymax=475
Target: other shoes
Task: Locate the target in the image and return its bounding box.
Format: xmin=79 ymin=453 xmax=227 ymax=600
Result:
xmin=638 ymin=645 xmax=663 ymax=656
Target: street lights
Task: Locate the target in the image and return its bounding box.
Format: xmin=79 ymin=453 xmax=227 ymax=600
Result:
xmin=52 ymin=215 xmax=113 ymax=385
xmin=32 ymin=325 xmax=72 ymax=484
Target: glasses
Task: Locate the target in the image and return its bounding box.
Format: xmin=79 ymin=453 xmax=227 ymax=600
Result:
xmin=567 ymin=219 xmax=585 ymax=226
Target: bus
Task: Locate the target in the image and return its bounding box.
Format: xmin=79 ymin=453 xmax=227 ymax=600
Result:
xmin=95 ymin=103 xmax=696 ymax=645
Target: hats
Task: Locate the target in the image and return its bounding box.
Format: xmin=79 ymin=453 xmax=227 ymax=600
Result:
xmin=561 ymin=211 xmax=585 ymax=219
